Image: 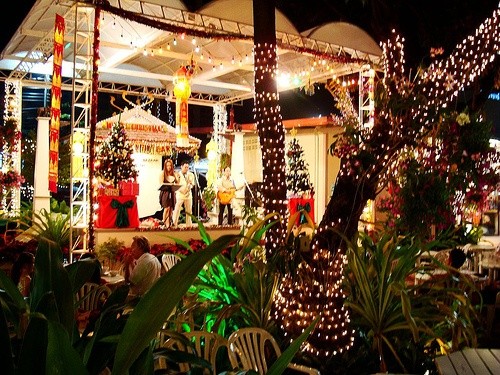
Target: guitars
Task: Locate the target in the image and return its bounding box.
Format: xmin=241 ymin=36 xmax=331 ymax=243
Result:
xmin=217 ymin=182 xmax=249 ymax=205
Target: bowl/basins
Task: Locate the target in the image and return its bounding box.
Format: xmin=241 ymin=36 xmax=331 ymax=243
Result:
xmin=110 ymin=271 xmax=117 ymax=277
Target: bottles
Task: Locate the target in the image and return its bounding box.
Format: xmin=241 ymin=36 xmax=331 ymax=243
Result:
xmin=63 ymin=258 xmax=68 ymax=267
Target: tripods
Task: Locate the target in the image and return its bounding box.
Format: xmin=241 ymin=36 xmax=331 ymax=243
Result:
xmin=158 ymin=184 xmax=182 ymax=227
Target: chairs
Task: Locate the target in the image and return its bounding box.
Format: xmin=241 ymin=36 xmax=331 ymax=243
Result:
xmin=227 ymin=326 xmax=321 ymax=375
xmin=159 ymin=328 xmax=229 ymax=375
xmin=161 ymin=254 xmax=182 ymax=274
xmin=442 ymin=272 xmax=474 ymax=309
xmin=77 ymin=280 xmax=111 ymax=322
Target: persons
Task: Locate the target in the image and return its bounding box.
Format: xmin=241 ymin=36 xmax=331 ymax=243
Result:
xmin=81 ymin=253 xmax=112 ymax=312
xmin=159 ymin=159 xmax=181 ymax=228
xmin=122 ymin=236 xmax=161 ymax=295
xmin=217 ymin=166 xmax=243 ymax=226
xmin=427 ymin=249 xmax=466 ymax=282
xmin=11 ymin=252 xmax=35 ymax=298
xmin=172 ymin=160 xmax=195 ymax=229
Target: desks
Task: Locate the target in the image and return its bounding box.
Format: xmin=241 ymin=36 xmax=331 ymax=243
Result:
xmin=416 ymin=267 xmax=491 ymax=299
xmin=102 ymin=271 xmax=126 ymax=286
